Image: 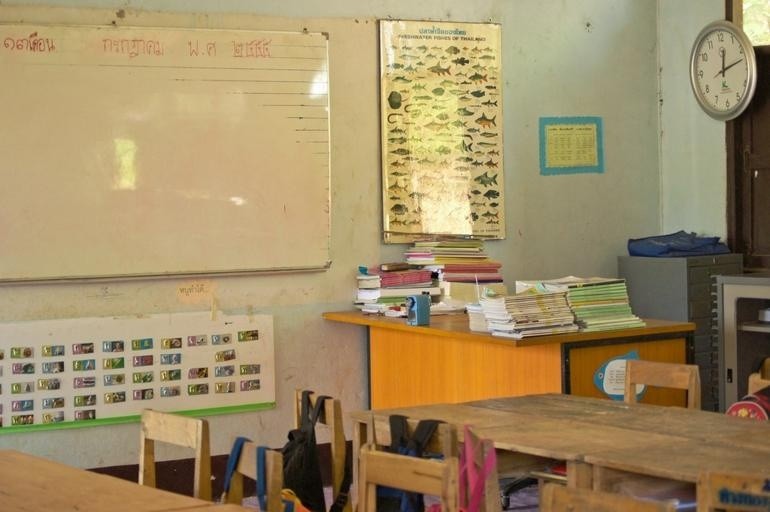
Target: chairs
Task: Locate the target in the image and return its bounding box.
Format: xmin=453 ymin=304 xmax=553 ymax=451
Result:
xmin=625 ymin=361 xmax=699 ymax=413
xmin=293 ymin=387 xmax=347 ymax=512
xmin=539 ymin=484 xmax=679 ymax=511
xmin=464 ymin=423 xmax=502 ymax=512
xmin=350 ymin=412 xmax=457 ymax=511
xmin=357 ymin=445 xmax=459 ymax=511
xmin=138 ymin=408 xmax=212 ymax=501
xmin=221 ymin=436 xmax=282 ymax=512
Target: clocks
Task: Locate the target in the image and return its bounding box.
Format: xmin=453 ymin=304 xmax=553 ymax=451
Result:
xmin=690 ymin=21 xmax=756 ymax=122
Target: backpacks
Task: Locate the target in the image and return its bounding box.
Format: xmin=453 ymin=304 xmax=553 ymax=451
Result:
xmin=376 ymin=415 xmax=445 ymax=511
xmin=723 ymin=392 xmax=770 ymax=422
xmin=281 ymin=391 xmax=350 ymax=512
xmin=221 ymin=436 xmax=310 ymax=512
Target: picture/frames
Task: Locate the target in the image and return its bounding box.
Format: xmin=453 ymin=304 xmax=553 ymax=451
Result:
xmin=376 ymin=18 xmax=506 ymax=244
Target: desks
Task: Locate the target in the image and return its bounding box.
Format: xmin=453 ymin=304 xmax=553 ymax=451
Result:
xmin=0 ymin=449 xmax=214 ymax=512
xmin=323 ymin=311 xmax=696 ymax=411
xmin=347 ymin=393 xmax=770 ymax=512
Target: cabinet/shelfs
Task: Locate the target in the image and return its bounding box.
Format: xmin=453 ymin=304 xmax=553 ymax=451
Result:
xmin=618 ymin=252 xmax=743 ymax=413
xmin=711 ymin=273 xmax=770 ymax=413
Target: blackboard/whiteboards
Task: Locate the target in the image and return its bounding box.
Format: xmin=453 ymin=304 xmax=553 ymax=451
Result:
xmin=0 ymin=22 xmax=333 ymax=284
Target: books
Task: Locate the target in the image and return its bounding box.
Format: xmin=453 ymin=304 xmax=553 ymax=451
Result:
xmin=354 ymin=271 xmax=463 ymax=319
xmin=402 ymin=238 xmax=508 ymax=311
xmin=515 ymin=276 xmax=646 ymax=333
xmin=466 ymin=283 xmax=579 ymax=338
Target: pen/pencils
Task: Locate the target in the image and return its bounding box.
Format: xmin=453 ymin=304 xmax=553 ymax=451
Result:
xmin=474 ymin=273 xmax=480 ymax=300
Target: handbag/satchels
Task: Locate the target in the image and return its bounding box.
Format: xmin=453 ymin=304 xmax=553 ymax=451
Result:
xmin=628 ymin=230 xmax=731 ymax=256
